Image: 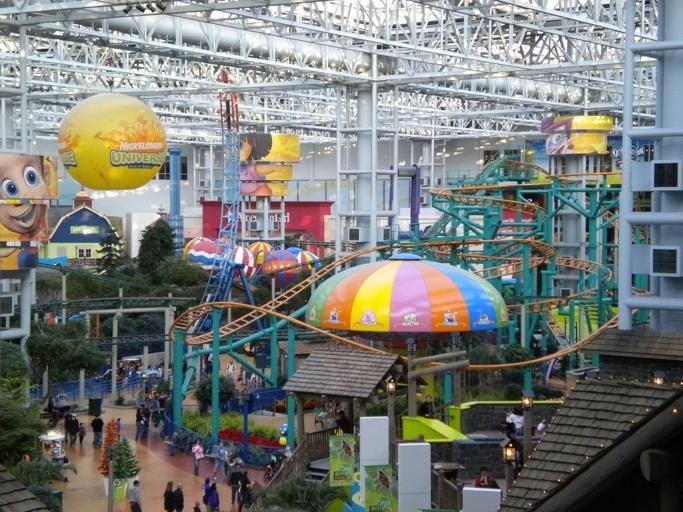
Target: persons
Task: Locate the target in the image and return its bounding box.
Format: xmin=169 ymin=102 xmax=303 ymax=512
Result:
xmin=497 ymin=405 xmax=549 ymax=437
xmin=117 ymin=357 xmax=290 ymax=512
xmin=419 ymin=396 xmax=434 ymax=419
xmin=42 ymin=392 xmax=104 ymax=464
xmin=476 ymin=467 xmax=499 ymax=489
xmin=323 ymin=397 xmax=349 ymax=434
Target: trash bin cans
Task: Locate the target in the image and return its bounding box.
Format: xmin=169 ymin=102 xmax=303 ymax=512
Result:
xmin=88 ymin=397 xmax=101 ymax=416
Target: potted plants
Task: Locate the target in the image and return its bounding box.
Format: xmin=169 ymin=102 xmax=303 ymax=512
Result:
xmin=99 ymin=417 xmax=142 ymax=503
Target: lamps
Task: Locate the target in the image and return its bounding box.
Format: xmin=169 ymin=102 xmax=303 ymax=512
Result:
xmin=385 ymin=376 xmax=396 ymax=395
xmin=122 ymin=1 xmax=167 ymax=14
xmin=501 ymin=439 xmax=518 ymax=462
xmin=652 ymin=376 xmax=664 ymax=384
xmin=520 ymin=391 xmax=535 ymax=409
